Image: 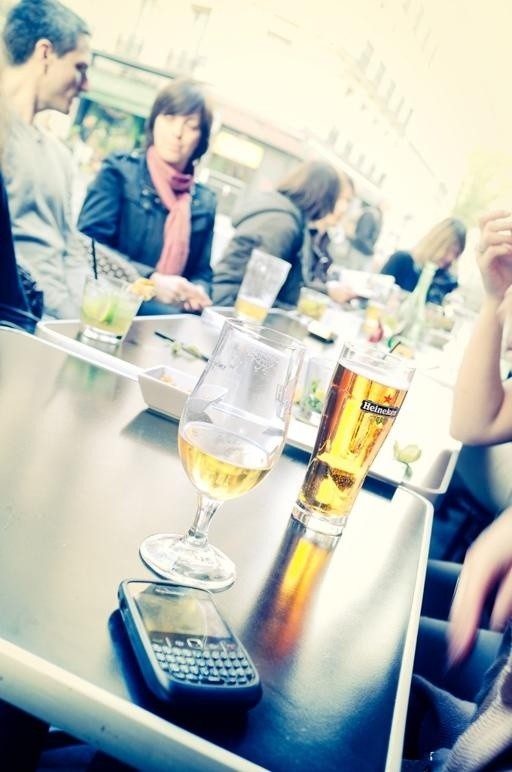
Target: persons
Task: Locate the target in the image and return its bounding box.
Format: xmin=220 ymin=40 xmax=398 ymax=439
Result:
xmin=379 ymin=217 xmax=465 ymax=308
xmin=77 ymin=75 xmax=217 ymax=317
xmin=346 ymin=199 xmax=379 ymax=271
xmin=403 ymin=207 xmax=511 ymax=772
xmin=1 ymin=1 xmax=94 ymax=333
xmin=211 ymin=160 xmax=360 ymax=313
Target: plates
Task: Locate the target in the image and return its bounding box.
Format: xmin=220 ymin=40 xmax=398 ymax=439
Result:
xmin=138 ymin=364 xmax=408 ymax=485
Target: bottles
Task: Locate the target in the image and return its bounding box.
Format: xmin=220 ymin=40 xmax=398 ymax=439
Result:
xmin=386 ymin=262 xmax=438 ymax=348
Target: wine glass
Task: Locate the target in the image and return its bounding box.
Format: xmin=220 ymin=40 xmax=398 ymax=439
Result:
xmin=139 ymin=319 xmax=306 ymax=592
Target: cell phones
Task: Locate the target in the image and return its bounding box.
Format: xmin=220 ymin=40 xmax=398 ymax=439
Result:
xmin=117 ymin=578 xmax=261 ymax=710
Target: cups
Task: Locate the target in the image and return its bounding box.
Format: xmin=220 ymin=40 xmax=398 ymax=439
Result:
xmin=232 ymin=248 xmax=332 ymax=325
xmin=244 ymin=521 xmax=345 ymax=651
xmin=360 ymin=297 xmax=384 ymax=339
xmin=291 ymin=339 xmax=416 ymax=536
xmin=80 ymin=272 xmax=145 ymax=346
